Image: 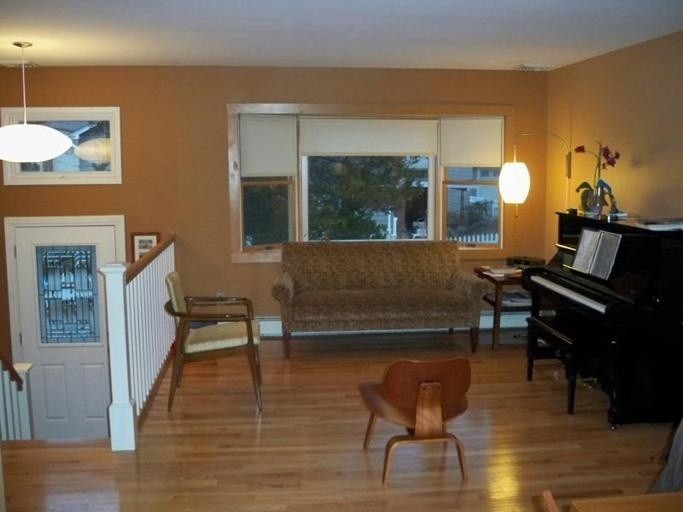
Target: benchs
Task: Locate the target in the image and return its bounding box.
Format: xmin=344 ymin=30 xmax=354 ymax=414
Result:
xmin=525 ymin=316 xmax=605 ymax=414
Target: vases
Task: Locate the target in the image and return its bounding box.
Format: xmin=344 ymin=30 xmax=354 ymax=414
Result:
xmin=581 ymin=188 xmax=604 ymax=215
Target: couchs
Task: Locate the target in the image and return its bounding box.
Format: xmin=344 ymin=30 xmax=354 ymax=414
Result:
xmin=271 ymin=239 xmax=495 ymax=358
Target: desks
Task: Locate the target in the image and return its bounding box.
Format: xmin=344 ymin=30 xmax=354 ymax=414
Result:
xmin=570 ymin=492 xmax=683 ymax=512
xmin=470 ymin=265 xmax=553 ymax=353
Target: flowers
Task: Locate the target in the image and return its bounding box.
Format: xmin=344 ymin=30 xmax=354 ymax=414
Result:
xmin=574 ymin=138 xmax=620 ymax=212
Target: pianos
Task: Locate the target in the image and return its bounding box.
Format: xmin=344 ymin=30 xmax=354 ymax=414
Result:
xmin=522 ymin=209 xmax=683 ymax=430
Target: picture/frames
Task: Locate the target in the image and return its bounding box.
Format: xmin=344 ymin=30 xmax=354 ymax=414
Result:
xmin=0 ymin=107 xmax=122 ymax=186
xmin=132 ymin=231 xmax=160 ymax=264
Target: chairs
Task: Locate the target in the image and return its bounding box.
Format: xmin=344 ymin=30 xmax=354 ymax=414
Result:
xmin=542 ymin=489 xmax=560 ymax=511
xmin=164 ymin=272 xmax=263 ymax=413
xmin=358 ymin=358 xmax=472 ymax=486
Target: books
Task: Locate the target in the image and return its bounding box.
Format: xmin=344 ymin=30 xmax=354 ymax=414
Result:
xmin=636 ymin=221 xmax=683 ymax=232
xmin=572 ymin=227 xmax=623 ymax=282
xmin=482 ymin=268 xmax=532 ymax=306
